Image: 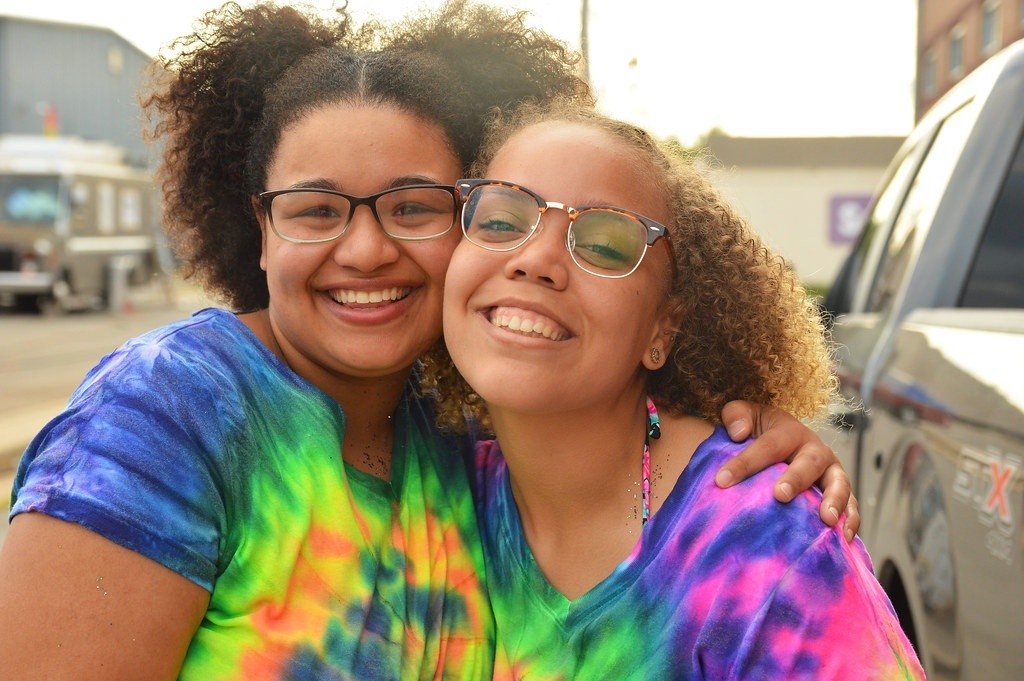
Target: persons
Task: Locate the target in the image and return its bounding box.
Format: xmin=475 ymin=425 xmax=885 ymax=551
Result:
xmin=0 ymin=0 xmax=860 ymax=681
xmin=421 ymin=94 xmax=926 ymax=681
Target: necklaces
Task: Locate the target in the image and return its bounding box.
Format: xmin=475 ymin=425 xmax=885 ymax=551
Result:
xmin=642 ymin=397 xmax=661 ymax=527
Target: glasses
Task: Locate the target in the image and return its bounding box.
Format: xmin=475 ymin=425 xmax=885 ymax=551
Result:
xmin=260 ymin=184 xmax=467 ymax=243
xmin=454 ymin=178 xmax=679 ymax=279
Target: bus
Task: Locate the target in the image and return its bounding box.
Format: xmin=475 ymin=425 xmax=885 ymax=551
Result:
xmin=0 ymin=160 xmax=157 ymax=314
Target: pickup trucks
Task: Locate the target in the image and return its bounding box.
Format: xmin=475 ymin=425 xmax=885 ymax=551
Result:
xmin=806 ymin=39 xmax=1024 ymax=680
